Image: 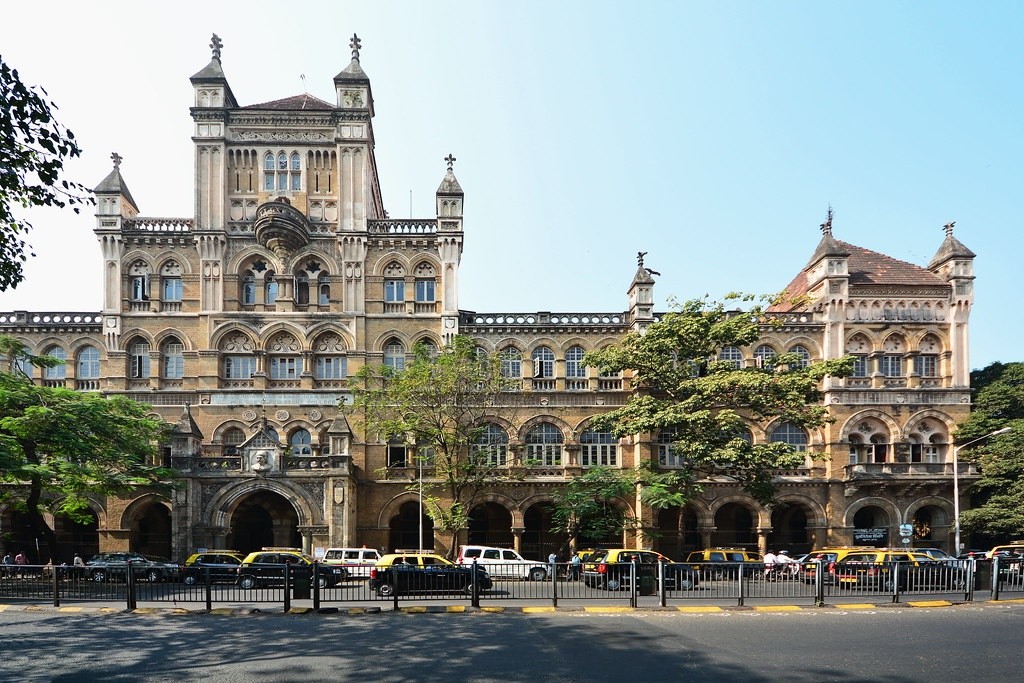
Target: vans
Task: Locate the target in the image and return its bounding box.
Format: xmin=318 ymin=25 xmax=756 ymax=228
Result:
xmin=684 ymin=546 xmax=766 ymax=582
xmin=322 ymin=545 xmax=383 ymax=578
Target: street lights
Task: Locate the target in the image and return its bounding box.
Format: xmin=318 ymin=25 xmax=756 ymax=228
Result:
xmin=397 ymin=432 xmax=423 ymax=554
xmin=953 ymin=425 xmax=1012 ymax=555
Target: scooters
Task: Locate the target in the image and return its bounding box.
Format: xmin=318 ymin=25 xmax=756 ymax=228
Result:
xmin=774 ymin=563 xmax=794 ymax=579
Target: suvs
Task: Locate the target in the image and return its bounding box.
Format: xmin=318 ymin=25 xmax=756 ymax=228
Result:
xmin=456 ymin=544 xmax=553 ymax=580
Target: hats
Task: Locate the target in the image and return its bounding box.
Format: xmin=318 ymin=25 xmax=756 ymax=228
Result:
xmin=780 ymin=550 xmax=789 ymax=554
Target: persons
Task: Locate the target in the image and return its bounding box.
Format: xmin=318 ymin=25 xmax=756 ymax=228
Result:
xmin=548 ymin=550 xmax=581 ymax=581
xmin=4 ymin=550 xmax=84 ymax=578
xmin=763 ymin=549 xmax=800 ymax=572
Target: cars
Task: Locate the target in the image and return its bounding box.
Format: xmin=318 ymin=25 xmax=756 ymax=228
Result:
xmin=181 ymin=552 xmax=241 ymax=586
xmin=84 ymin=551 xmax=187 ymax=583
xmin=235 ymin=551 xmax=348 ymax=591
xmin=790 ymin=541 xmax=1024 ymax=590
xmin=581 ymin=547 xmax=701 ymax=592
xmin=369 ymin=554 xmax=494 ymax=597
